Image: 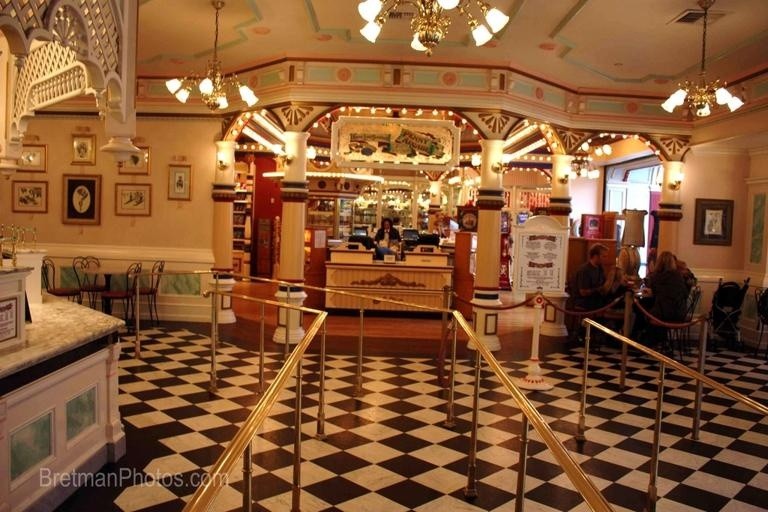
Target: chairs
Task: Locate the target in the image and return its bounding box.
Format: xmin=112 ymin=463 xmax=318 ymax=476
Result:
xmin=565 ymin=260 xmax=767 ymax=362
xmin=39 ymin=254 xmax=165 ymax=333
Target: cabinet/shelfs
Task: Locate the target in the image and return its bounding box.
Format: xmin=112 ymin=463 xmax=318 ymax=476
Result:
xmin=302 ymin=224 xmax=328 ymax=316
xmin=232 ymin=188 xmax=252 ymax=277
xmin=566 ymin=237 xmax=617 ymax=285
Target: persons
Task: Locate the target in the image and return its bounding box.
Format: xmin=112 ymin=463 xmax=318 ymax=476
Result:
xmin=177 ymin=175 xmax=183 ymax=192
xmin=569 ymin=244 xmax=696 ymax=348
xmin=374 ymin=217 xmax=400 ymax=241
xmin=76 ymin=188 xmax=87 ymax=213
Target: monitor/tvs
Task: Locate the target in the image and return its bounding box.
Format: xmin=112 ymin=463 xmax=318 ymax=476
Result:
xmin=355 ymin=228 xmax=367 ymax=237
xmin=404 ymin=230 xmax=419 ymax=241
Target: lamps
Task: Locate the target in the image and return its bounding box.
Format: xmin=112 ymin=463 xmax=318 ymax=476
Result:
xmin=657 ymin=0 xmax=743 ymax=119
xmin=356 ymin=0 xmax=509 ymax=58
xmin=164 ymin=0 xmax=264 ymax=109
xmin=569 ymin=141 xmax=613 ymax=181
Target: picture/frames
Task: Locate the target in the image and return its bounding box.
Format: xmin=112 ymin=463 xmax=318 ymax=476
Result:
xmin=692 ymin=197 xmax=733 ymax=246
xmin=166 ymin=160 xmax=192 ymax=200
xmin=68 ymin=133 xmax=97 ymax=165
xmin=14 ymin=140 xmax=48 ymax=174
xmin=10 ymin=178 xmax=50 ymax=214
xmin=60 ymin=173 xmax=102 ymax=225
xmin=116 ymin=142 xmax=151 ymax=176
xmin=114 ymin=181 xmax=152 ymax=218
